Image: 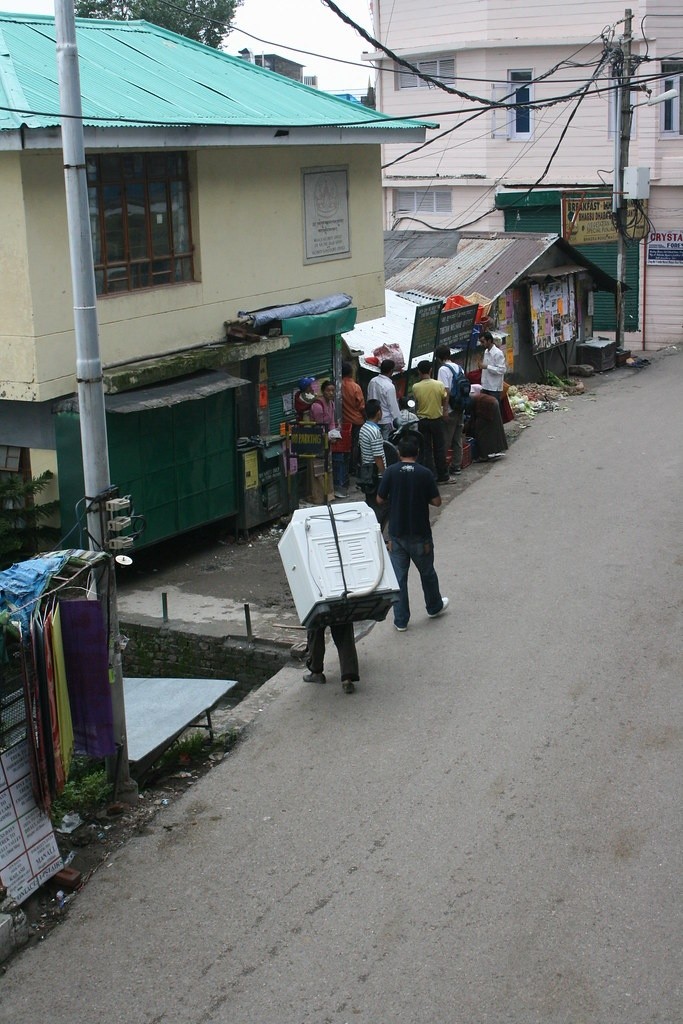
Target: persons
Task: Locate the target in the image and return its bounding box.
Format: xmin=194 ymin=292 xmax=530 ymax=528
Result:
xmin=413 ymin=361 xmax=456 ymax=484
xmin=359 ymin=399 xmax=388 ymax=533
xmin=302 ymin=621 xmax=359 ymax=693
xmin=435 ymin=345 xmax=470 ymax=475
xmin=377 ymin=436 xmax=450 ymax=631
xmin=294 ymin=377 xmax=341 ymax=472
xmin=464 ymin=332 xmax=506 ymax=462
xmin=343 ymin=365 xmax=365 ymax=475
xmin=368 ymin=360 xmax=400 ymax=439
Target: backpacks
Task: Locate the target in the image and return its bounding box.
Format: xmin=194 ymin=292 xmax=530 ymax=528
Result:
xmin=442 ymin=363 xmax=472 ymax=414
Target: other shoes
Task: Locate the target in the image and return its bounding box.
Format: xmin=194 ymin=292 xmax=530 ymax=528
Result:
xmin=303 ymin=673 xmax=326 ymax=684
xmin=335 ymin=491 xmax=350 ymax=498
xmin=473 ymin=457 xmax=488 ymax=463
xmin=437 ymin=476 xmax=456 ymax=485
xmin=343 ymin=682 xmax=355 ymax=693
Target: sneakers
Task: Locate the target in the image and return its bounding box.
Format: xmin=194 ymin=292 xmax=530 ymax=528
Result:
xmin=428 ymin=596 xmax=450 ymax=618
xmin=393 ymin=624 xmax=407 ymax=631
xmin=449 ymin=464 xmax=461 ymax=474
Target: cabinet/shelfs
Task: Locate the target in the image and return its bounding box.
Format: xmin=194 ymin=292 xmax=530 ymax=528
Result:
xmin=576 ymin=341 xmax=616 ymax=372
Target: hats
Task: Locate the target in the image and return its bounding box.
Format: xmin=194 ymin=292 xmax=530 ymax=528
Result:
xmin=298 ymin=376 xmax=316 ymax=392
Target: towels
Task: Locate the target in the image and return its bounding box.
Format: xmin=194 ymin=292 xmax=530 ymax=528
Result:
xmin=21 ymin=599 xmax=114 ymax=814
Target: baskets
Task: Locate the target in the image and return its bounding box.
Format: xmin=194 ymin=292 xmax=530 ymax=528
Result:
xmin=331 ymin=423 xmax=352 ymax=452
xmin=333 ymin=451 xmax=350 ymax=462
xmin=333 ymin=462 xmax=350 ymax=486
xmin=446 ymin=443 xmax=471 ymax=468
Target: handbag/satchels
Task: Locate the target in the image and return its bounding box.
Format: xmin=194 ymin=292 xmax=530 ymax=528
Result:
xmin=373 ymin=343 xmax=405 ymax=370
xmin=502 ymin=391 xmax=514 ymax=423
xmin=355 ymin=462 xmax=378 ymax=494
